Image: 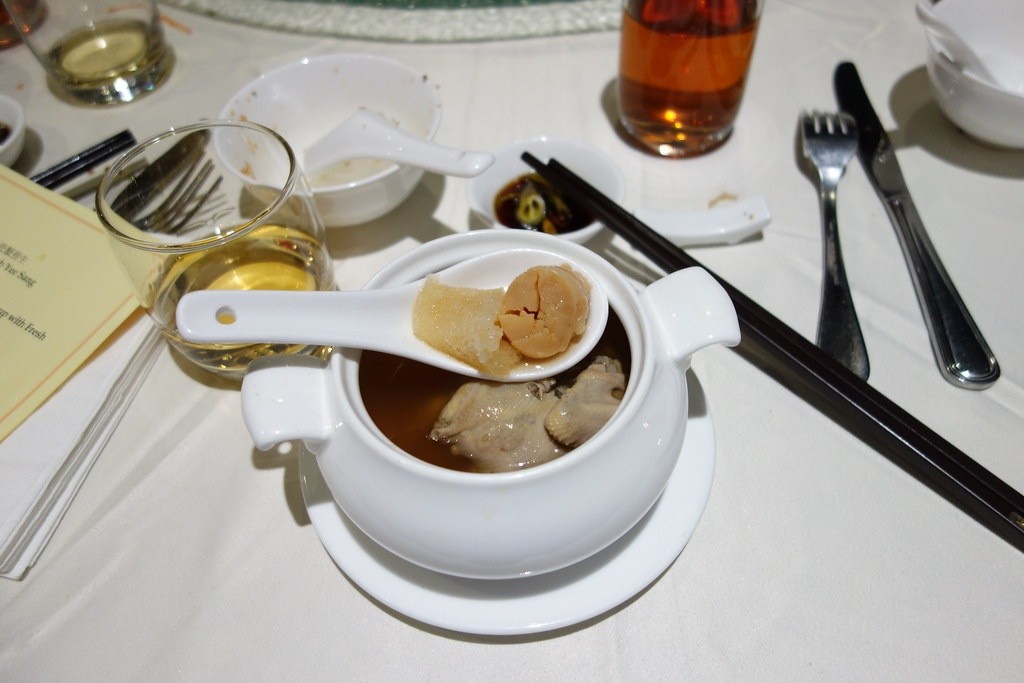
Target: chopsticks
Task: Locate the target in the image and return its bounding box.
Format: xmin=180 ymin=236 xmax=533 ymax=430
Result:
xmin=520 ymin=151 xmax=1024 ymax=550
xmin=30 ymin=128 xmax=137 ymax=190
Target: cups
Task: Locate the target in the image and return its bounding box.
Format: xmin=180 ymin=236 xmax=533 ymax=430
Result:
xmin=95 ymin=117 xmax=340 ymax=384
xmin=0 ymin=0 xmax=174 ymax=107
xmin=615 ymin=0 xmax=766 ymax=156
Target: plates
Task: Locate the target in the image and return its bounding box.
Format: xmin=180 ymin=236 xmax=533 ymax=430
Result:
xmin=297 ymin=368 xmax=717 ymax=639
xmin=619 ymin=156 xmax=774 ymax=245
xmin=467 ymin=140 xmax=625 ymax=245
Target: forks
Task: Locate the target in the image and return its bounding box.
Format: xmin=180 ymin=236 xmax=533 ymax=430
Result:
xmin=132 ymin=150 xmax=223 ymax=233
xmin=799 ymin=106 xmax=870 ymax=384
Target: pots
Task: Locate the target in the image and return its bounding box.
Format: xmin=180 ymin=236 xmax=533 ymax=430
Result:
xmin=238 ymin=226 xmax=742 ymax=581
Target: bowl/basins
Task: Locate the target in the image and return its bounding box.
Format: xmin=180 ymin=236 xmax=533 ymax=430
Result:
xmin=210 ymin=53 xmax=442 ymax=226
xmin=926 ymin=0 xmax=1024 ymax=152
xmin=0 ymin=94 xmax=25 ymax=169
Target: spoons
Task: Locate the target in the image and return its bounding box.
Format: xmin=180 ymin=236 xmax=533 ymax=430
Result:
xmin=175 ymin=246 xmax=610 ymax=381
xmin=107 ymin=129 xmax=211 ymax=216
xmin=301 ymin=110 xmax=494 ymax=178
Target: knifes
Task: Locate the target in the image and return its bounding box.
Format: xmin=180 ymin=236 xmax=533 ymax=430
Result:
xmin=834 ymin=61 xmax=1002 ymax=390
